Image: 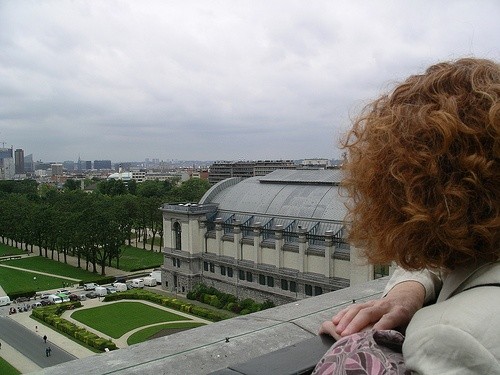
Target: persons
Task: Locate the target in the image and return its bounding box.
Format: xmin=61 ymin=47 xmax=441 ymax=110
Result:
xmin=318 ymin=58 xmax=500 ymax=375
xmin=43 ymin=335 xmax=52 ymax=357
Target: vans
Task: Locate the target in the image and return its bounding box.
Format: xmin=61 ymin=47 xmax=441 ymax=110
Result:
xmin=83 ymin=283 xmax=99 ymax=291
xmin=33 ymin=269 xmax=162 ymax=308
xmin=0 ymin=295 xmax=11 ymax=307
